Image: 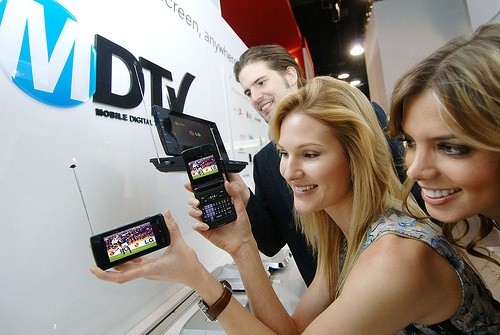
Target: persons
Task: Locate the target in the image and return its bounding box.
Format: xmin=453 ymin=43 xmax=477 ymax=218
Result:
xmin=382 ymin=22 xmax=498 ymax=266
xmin=116 ymin=234 xmax=131 ymax=254
xmin=88 ymin=74 xmax=500 ymax=335
xmin=190 ymin=43 xmax=448 ymax=290
xmin=195 ymin=161 xmax=204 ymax=175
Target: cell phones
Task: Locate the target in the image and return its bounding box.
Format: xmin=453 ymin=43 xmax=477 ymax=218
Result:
xmin=181 ymin=143 xmax=238 ymax=230
xmin=90 ymin=212 xmax=170 ymax=271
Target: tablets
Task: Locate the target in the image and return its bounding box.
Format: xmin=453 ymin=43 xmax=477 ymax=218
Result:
xmin=152 ymin=105 xmax=229 ymax=161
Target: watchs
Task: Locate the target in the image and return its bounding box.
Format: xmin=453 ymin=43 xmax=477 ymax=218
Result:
xmin=197 ymin=279 xmax=233 ymax=321
